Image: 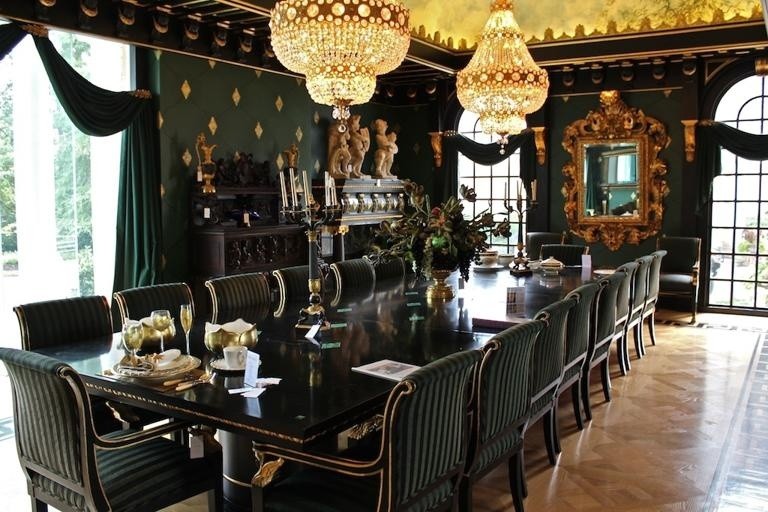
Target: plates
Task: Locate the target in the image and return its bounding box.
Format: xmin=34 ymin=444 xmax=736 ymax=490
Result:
xmin=210 ymin=358 xmax=261 ymax=371
xmin=473 ymin=265 xmax=505 ymax=271
xmin=593 ymin=268 xmax=615 ymax=275
xmin=112 ymin=353 xmax=202 ymax=381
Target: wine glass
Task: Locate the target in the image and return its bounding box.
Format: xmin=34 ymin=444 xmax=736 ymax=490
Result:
xmin=123 ymin=320 xmax=144 ymax=365
xmin=150 ymin=310 xmax=172 ymax=353
xmin=179 ymin=303 xmax=193 ymax=355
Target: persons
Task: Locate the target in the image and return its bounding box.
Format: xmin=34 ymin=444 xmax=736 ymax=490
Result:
xmin=348 ymin=115 xmax=372 ymax=179
xmin=372 ymin=119 xmax=398 ymax=180
xmin=328 ymin=113 xmax=350 ymax=179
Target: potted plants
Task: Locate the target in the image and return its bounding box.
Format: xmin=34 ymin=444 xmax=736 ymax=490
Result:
xmin=276 ymin=161 xmax=345 ymax=210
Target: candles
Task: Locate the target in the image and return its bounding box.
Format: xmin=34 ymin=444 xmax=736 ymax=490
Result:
xmin=501 ymin=176 xmax=541 ymax=203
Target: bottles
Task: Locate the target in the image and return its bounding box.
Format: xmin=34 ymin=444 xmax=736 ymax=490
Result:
xmin=540 ymin=255 xmax=565 ymax=272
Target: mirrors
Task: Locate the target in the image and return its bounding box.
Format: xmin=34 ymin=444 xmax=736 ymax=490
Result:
xmin=560 ymin=89 xmax=673 ymax=252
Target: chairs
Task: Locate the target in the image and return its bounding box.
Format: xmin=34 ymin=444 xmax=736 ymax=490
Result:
xmin=117 ymin=282 xmax=196 ymax=329
xmin=535 ymin=280 xmax=602 ymax=466
xmin=12 ymin=295 xmax=114 ymax=350
xmin=273 ymin=255 xmax=407 ymax=315
xmin=1 ymin=347 xmax=223 ymax=512
xmin=251 ymin=321 xmax=551 ymax=512
xmin=523 ymin=231 xmax=569 ymax=260
xmin=206 ymin=272 xmax=271 ymax=320
xmin=647 ymin=235 xmax=704 ymax=326
xmin=539 ymin=245 xmax=587 ymax=265
xmin=582 ymin=250 xmax=667 ymax=419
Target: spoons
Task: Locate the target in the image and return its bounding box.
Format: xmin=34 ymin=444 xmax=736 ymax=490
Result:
xmin=162 ymin=372 xmax=212 ymax=391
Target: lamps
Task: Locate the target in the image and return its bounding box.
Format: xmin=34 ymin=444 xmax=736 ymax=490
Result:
xmin=531 ymin=125 xmax=547 ymax=169
xmin=681 ymin=120 xmax=698 ymax=163
xmin=428 ymin=131 xmax=444 ymax=168
xmin=266 ymin=0 xmax=413 ymax=133
xmin=451 ymin=0 xmax=551 ymax=159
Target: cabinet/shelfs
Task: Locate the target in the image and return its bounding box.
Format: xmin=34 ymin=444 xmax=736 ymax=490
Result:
xmin=185 ymin=183 xmax=309 ymax=281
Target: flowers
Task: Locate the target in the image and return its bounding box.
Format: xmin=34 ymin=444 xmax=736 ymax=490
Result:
xmin=363 ymin=192 xmax=517 ymax=283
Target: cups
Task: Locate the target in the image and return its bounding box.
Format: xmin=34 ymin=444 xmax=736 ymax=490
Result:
xmin=222 ymin=345 xmax=248 ymax=367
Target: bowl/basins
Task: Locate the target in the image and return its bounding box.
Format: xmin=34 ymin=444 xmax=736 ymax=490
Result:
xmin=204 ymin=318 xmax=257 ymax=355
